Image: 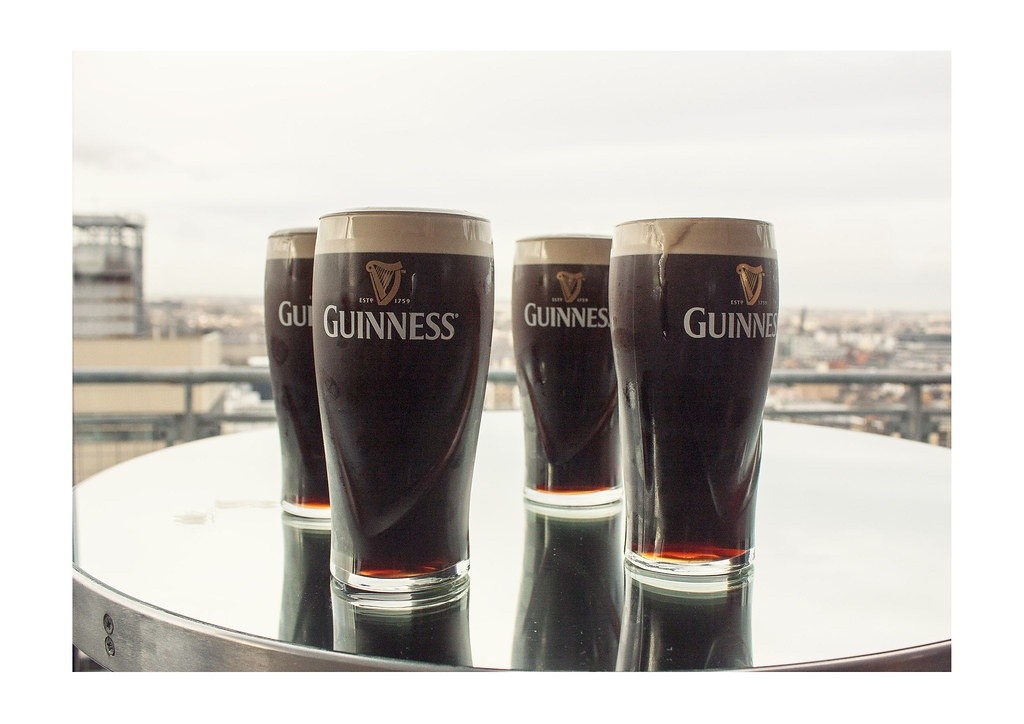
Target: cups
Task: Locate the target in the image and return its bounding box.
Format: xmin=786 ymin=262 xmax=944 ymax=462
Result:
xmin=608 ymin=217 xmax=779 ymax=580
xmin=510 ymin=235 xmax=625 ymax=508
xmin=311 ymin=208 xmax=495 ymax=594
xmin=264 ymin=226 xmax=331 ymax=519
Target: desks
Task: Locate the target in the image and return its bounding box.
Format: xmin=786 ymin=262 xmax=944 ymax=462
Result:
xmin=73 ymin=406 xmax=951 ymax=672
